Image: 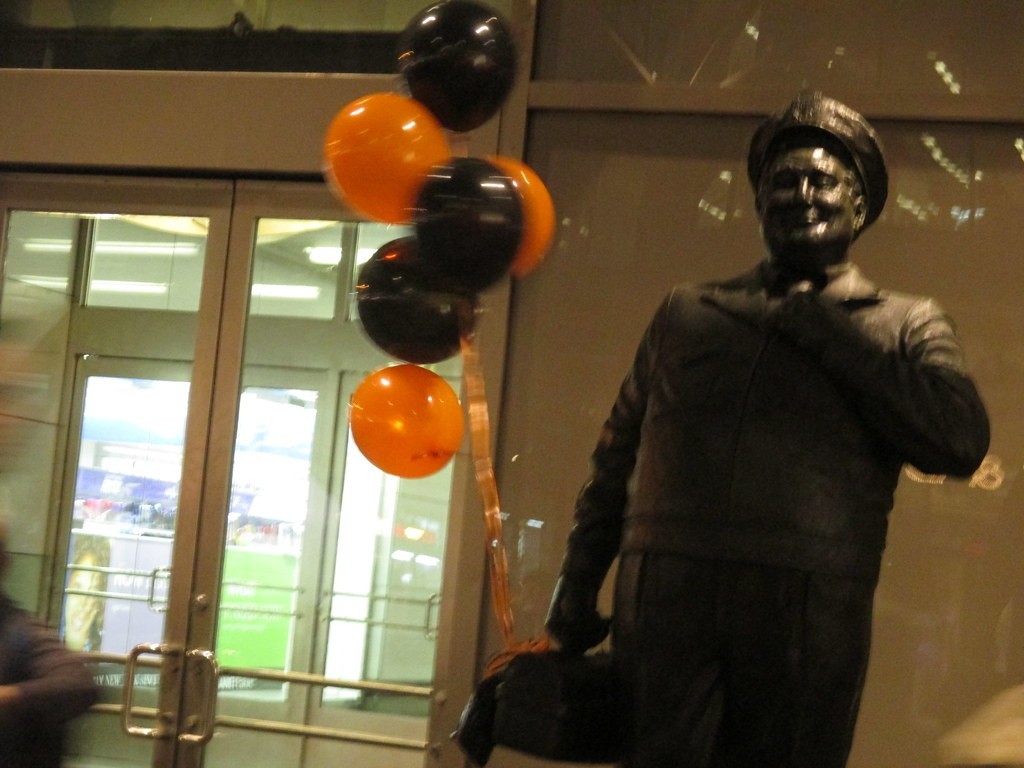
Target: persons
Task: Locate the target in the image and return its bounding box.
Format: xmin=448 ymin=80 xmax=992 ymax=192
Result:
xmin=453 ymin=89 xmax=990 ymax=768
xmin=59 ymin=536 xmax=110 ymax=654
xmin=0 ymin=521 xmax=97 ymax=768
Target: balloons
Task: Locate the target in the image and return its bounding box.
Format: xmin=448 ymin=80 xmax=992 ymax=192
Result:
xmin=412 ymin=153 xmax=553 ymax=296
xmin=349 ymin=363 xmax=463 ymax=479
xmin=355 ymin=236 xmax=478 ymax=364
xmin=325 ymin=93 xmax=449 ymax=224
xmin=401 ymin=0 xmax=515 ymax=133
